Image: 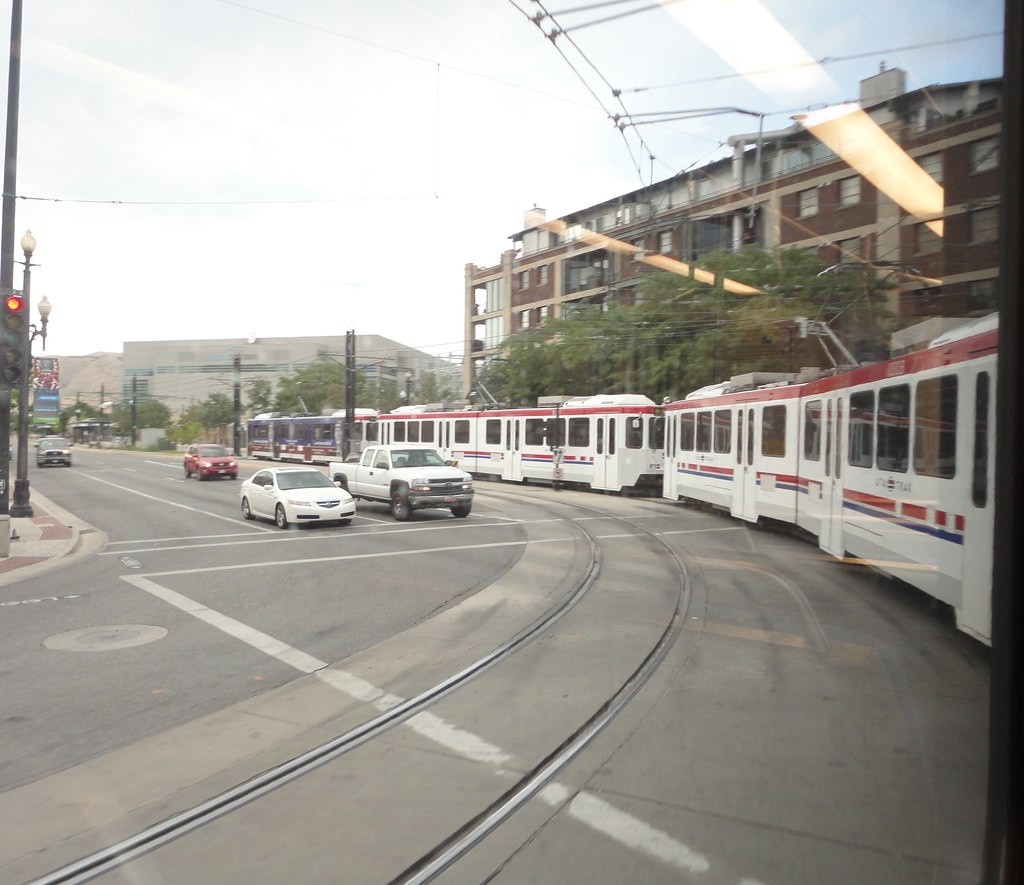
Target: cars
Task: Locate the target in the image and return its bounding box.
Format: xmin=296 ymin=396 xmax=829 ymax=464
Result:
xmin=183 ymin=444 xmax=239 ymax=481
xmin=241 ymin=467 xmax=356 ymax=530
xmin=9 ymin=444 xmax=12 ymax=460
xmin=34 ymin=437 xmax=74 ymax=469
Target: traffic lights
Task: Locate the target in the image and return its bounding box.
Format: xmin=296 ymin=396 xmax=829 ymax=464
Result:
xmin=0 ymin=294 xmax=24 ymax=383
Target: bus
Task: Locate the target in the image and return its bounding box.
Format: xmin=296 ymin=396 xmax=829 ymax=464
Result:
xmin=34 ymin=426 xmax=56 ymax=447
xmin=249 ymin=313 xmax=992 ymax=649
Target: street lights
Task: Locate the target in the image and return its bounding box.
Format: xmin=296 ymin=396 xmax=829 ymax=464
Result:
xmin=8 ymin=231 xmax=52 ymax=517
xmin=399 ymin=371 xmax=412 ymax=404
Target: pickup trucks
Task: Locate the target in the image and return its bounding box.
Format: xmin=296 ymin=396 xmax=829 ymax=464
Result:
xmin=328 ymin=444 xmax=474 ymax=521
